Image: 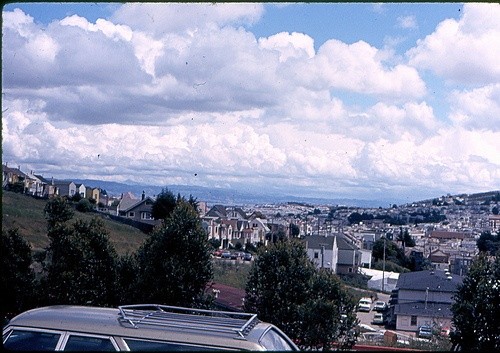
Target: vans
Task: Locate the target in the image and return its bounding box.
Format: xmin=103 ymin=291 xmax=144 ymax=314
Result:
xmin=2 ymin=304 xmax=299 ymax=352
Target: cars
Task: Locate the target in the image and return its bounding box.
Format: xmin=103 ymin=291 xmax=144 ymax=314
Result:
xmin=431 ymin=324 xmax=451 ymax=341
xmin=419 ymin=323 xmax=433 ymax=339
xmin=372 ymin=313 xmax=383 ymax=323
xmin=210 ymin=249 xmax=253 ymax=261
xmin=373 ymin=300 xmax=385 ymax=310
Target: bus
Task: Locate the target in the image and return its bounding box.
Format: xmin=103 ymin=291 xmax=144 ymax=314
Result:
xmin=358 ymin=297 xmax=372 ymax=311
